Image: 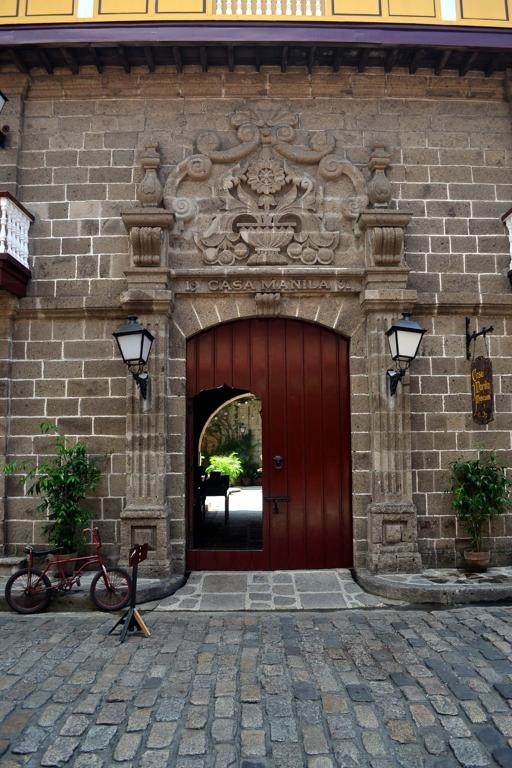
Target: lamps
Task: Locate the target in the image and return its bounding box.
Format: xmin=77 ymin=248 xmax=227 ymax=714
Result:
xmin=110 ymin=314 xmax=156 ymax=401
xmin=384 ymin=310 xmax=429 ymax=397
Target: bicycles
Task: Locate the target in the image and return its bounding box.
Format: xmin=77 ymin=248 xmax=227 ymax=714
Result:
xmin=4 ymin=526 xmax=132 ymax=614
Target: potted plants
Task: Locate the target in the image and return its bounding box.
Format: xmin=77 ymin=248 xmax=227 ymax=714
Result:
xmin=0 ymin=420 xmax=116 ymax=579
xmin=442 ymin=440 xmax=512 ymax=573
